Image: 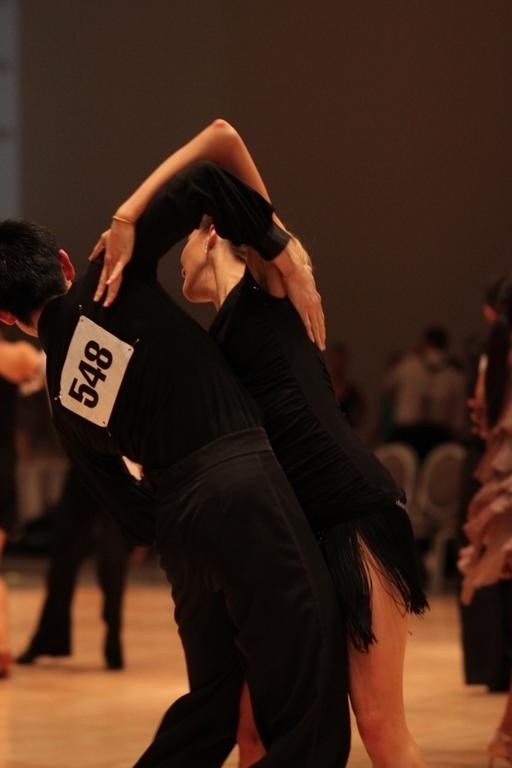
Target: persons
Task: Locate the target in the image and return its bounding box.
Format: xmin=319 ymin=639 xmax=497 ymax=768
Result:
xmin=0 ymin=154 xmax=352 ymax=768
xmin=322 ymin=264 xmax=510 ymax=766
xmin=15 ymin=445 xmax=129 ymax=675
xmin=86 ymin=118 xmax=428 ymax=768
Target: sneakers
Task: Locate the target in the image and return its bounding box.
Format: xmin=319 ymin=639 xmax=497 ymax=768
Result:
xmin=16 ymin=637 xmax=53 ymax=664
xmin=103 ymin=627 xmax=124 ymax=670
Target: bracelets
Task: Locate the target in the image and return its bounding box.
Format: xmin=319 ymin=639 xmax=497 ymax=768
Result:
xmin=111 ymin=213 xmax=138 ymax=228
